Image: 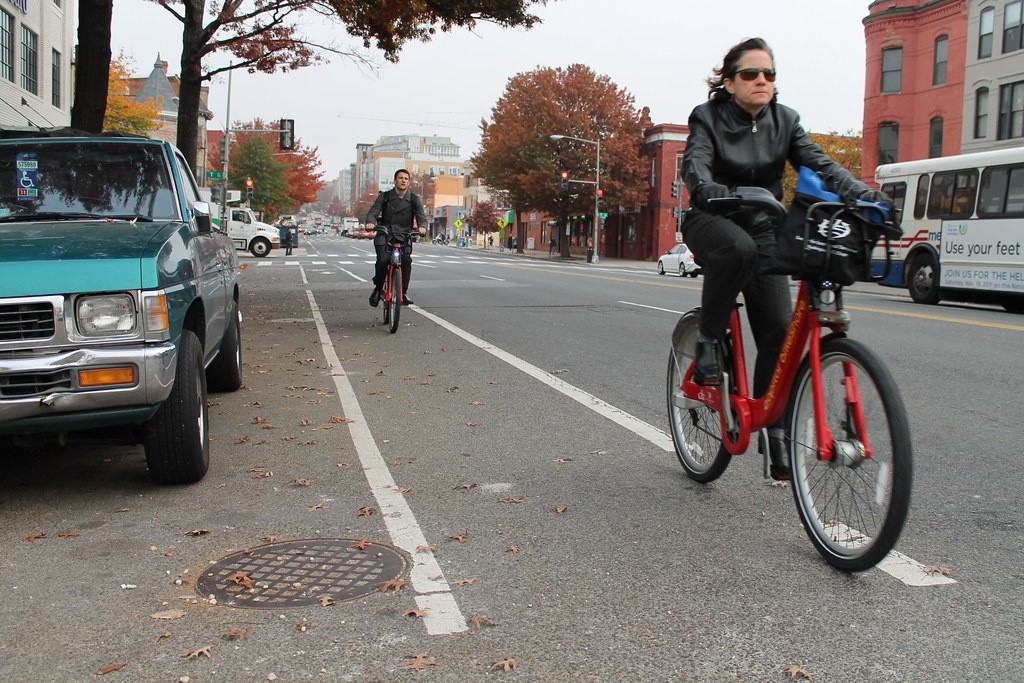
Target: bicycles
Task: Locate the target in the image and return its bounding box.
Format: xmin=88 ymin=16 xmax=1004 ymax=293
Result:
xmin=365 ymin=224 xmax=428 ymax=335
xmin=662 ymin=194 xmax=916 ymax=575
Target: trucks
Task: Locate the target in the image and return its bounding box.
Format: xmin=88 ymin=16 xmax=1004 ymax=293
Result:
xmin=341 ymin=217 xmax=359 ymax=236
xmin=208 ymin=203 xmax=281 ymax=258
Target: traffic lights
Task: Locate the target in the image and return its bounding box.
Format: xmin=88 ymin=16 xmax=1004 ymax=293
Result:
xmin=247 ymin=176 xmax=254 ymax=200
xmin=560 ymin=172 xmax=568 ymax=190
xmin=671 ymin=181 xmax=680 ymax=199
xmin=596 ymin=189 xmax=603 ymax=207
xmin=671 ymin=205 xmax=680 ymax=220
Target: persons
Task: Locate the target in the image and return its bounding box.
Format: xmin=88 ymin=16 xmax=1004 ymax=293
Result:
xmin=552 ymin=237 xmax=556 ymax=254
xmin=286 ymin=228 xmax=293 ymax=256
xmin=508 ymin=234 xmax=513 ymax=250
xmin=678 ymin=37 xmax=895 ymax=479
xmin=365 ymin=169 xmax=428 ymax=307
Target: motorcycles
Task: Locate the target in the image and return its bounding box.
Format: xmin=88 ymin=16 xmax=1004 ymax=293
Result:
xmin=432 ymin=233 xmax=450 ymax=246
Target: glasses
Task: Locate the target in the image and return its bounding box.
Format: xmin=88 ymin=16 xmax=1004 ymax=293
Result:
xmin=733 ymin=66 xmax=777 ymax=84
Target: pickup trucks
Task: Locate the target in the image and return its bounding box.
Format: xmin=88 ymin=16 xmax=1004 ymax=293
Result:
xmin=0 ymin=137 xmax=245 ymax=483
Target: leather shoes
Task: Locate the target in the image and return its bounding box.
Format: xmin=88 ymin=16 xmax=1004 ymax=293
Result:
xmin=402 ymin=293 xmax=414 ymax=305
xmin=693 ymin=339 xmax=723 ymax=386
xmin=368 ymin=288 xmax=382 ymax=307
xmin=757 ymin=432 xmax=795 ymax=481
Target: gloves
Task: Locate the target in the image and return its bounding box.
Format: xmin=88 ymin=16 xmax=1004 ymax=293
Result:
xmin=861 ymin=188 xmax=899 ymax=222
xmin=692 ymin=182 xmax=731 ymax=213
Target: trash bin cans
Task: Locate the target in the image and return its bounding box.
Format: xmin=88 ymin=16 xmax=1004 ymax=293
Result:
xmin=468 ymin=240 xmax=472 ymax=246
xmin=587 ymin=247 xmax=594 ymax=262
xmin=279 ymin=226 xmax=298 ymax=248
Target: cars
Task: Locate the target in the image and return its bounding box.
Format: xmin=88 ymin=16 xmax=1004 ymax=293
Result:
xmin=656 ymin=243 xmax=704 ymax=280
xmin=297 ymin=214 xmax=340 ymax=236
xmin=348 ymin=225 xmax=375 ymax=240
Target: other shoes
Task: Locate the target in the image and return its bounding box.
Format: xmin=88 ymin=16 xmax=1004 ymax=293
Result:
xmin=285 ymin=252 xmax=292 ymax=256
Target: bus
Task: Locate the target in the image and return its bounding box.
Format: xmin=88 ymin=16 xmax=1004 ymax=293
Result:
xmin=873 ymin=145 xmax=1024 ymax=301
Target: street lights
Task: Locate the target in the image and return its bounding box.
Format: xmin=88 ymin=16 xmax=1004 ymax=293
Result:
xmin=550 ymin=134 xmax=601 ymax=266
